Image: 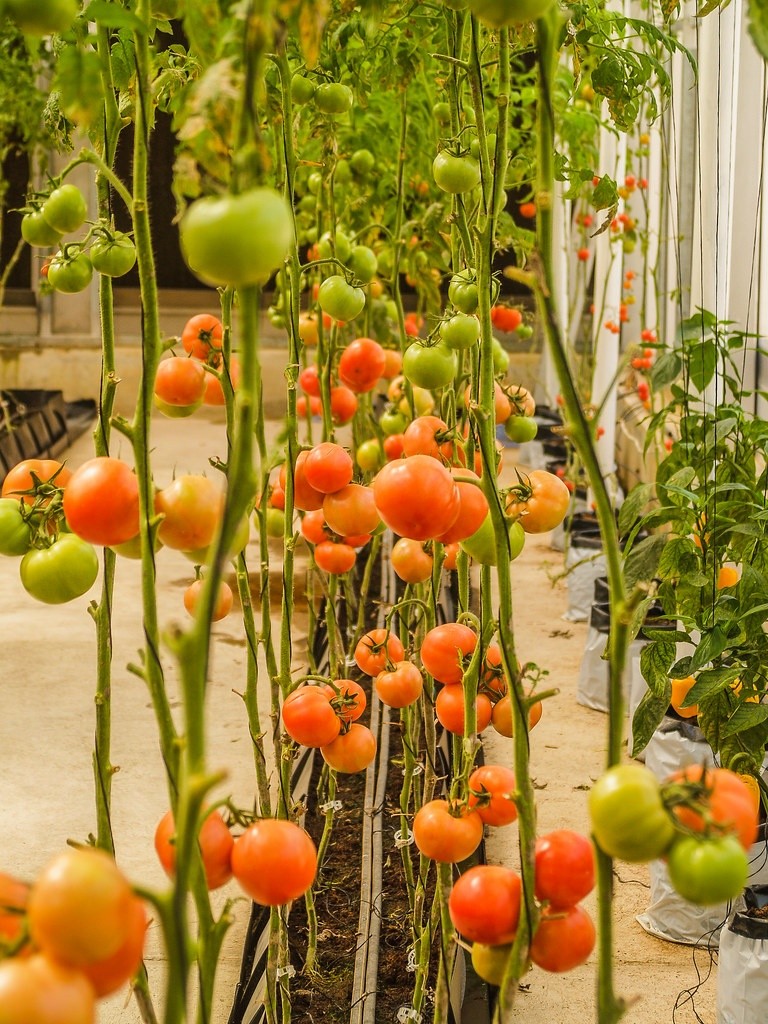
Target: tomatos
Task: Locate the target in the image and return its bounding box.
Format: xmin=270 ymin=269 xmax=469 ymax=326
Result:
xmin=0 ymin=76 xmax=768 ymax=1024
